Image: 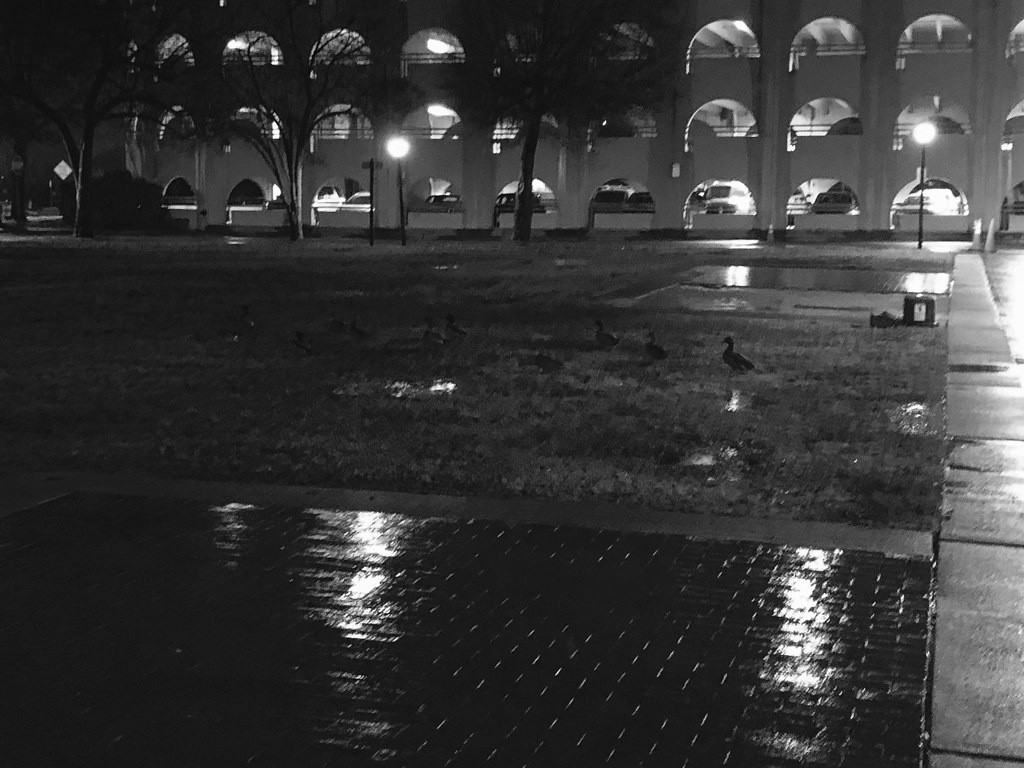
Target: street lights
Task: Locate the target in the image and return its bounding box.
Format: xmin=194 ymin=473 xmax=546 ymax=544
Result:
xmin=912 ymin=120 xmax=938 ymax=250
xmin=384 ymin=134 xmax=412 ymax=244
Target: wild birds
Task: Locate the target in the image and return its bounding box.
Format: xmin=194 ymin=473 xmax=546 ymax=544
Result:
xmin=722 ymin=337 xmax=763 ymax=377
xmin=422 ymin=313 xmax=468 ymax=345
xmin=594 ymin=318 xmax=620 ymax=347
xmin=241 ymin=305 xmax=370 ymax=355
xmin=646 ymin=333 xmax=669 ymax=364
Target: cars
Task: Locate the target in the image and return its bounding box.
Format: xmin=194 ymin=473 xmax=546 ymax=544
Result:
xmin=339 ymin=176 xmax=970 ymax=218
